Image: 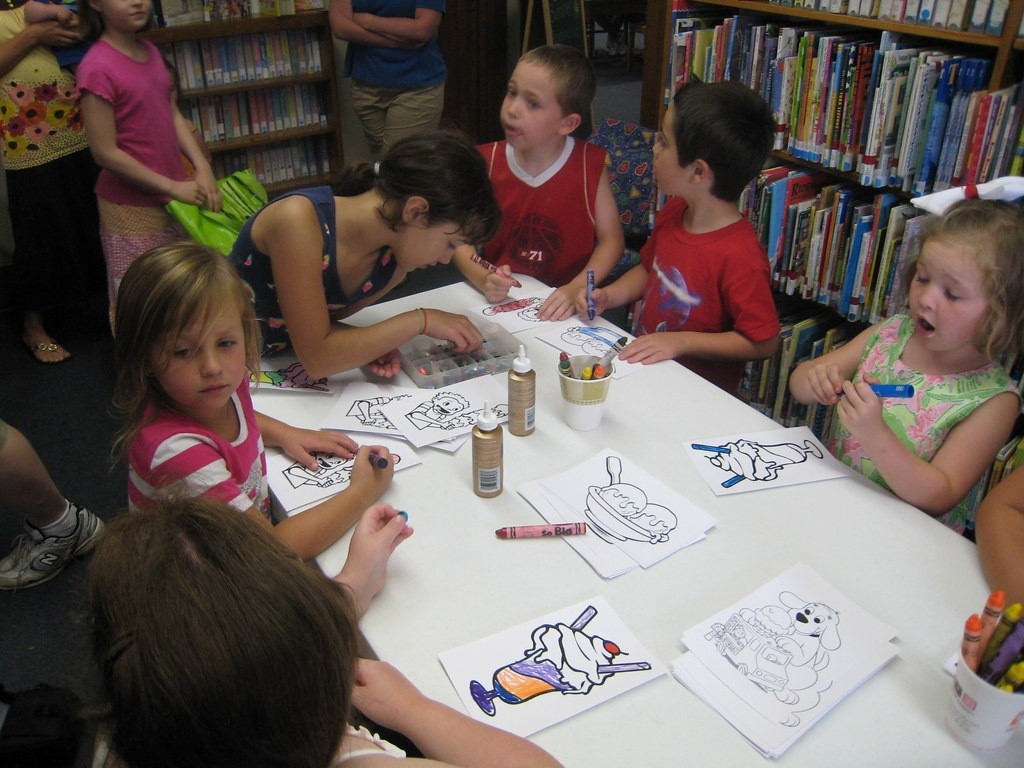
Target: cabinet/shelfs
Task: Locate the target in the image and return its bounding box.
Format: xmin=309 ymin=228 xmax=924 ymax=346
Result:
xmin=640 ymin=0 xmax=1024 ymax=200
xmin=137 ymin=9 xmax=343 ymax=193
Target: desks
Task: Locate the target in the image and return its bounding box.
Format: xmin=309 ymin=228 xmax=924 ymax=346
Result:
xmin=244 ymin=264 xmax=1024 ymax=768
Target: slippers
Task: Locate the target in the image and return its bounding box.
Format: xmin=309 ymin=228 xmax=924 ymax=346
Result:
xmin=23 ymin=333 xmax=71 ymax=365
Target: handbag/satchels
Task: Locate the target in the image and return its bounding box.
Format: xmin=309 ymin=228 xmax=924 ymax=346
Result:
xmin=165 ymin=167 xmax=267 ymax=254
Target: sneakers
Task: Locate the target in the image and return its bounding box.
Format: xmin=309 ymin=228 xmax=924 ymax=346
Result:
xmin=0 ymin=502 xmax=107 ymax=593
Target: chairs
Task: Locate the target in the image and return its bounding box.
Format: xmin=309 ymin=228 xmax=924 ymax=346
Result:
xmin=583 ymin=118 xmax=658 ymax=287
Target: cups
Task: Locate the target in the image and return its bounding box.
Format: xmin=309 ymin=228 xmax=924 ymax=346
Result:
xmin=947 ymin=650 xmax=1024 ymax=752
xmin=558 ymin=354 xmax=615 ymax=431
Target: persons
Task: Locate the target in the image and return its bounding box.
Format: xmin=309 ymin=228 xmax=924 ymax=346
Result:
xmin=0 ymin=0 xmax=103 ymax=367
xmin=68 ymin=465 xmax=568 ymax=768
xmin=0 ymin=420 xmax=109 ymax=592
xmin=452 ymin=44 xmax=624 ymax=322
xmin=329 ymin=0 xmax=448 ymax=158
xmin=106 ymin=240 xmax=394 ymax=564
xmin=227 ymin=119 xmax=504 ymax=382
xmin=974 ymin=464 xmax=1024 ymax=611
xmin=789 ymin=196 xmax=1024 ymax=535
xmin=574 ymin=80 xmax=780 ymax=403
xmin=77 ymin=0 xmax=224 ymax=342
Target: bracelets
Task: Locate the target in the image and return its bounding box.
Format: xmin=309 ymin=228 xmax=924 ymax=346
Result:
xmin=416 ymin=306 xmax=427 ymax=336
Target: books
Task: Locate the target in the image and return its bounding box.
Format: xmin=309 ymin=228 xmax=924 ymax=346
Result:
xmin=786 ymin=26 xmax=1024 ymax=196
xmin=183 ymin=86 xmax=328 ymax=144
xmin=207 ymin=143 xmax=287 ymax=184
xmin=736 ymin=161 xmax=854 ymax=306
xmin=154 ymin=0 xmax=323 ymax=93
xmin=830 ymin=192 xmax=930 ymax=324
xmin=664 ymin=0 xmax=799 ymax=126
xmin=283 ymin=136 xmax=330 ymax=181
xmin=767 ymin=0 xmax=1010 ymax=36
xmin=717 ymin=306 xmax=857 ymax=439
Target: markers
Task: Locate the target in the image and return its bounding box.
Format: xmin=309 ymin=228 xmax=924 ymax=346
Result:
xmin=834 ymin=384 xmax=914 ymax=399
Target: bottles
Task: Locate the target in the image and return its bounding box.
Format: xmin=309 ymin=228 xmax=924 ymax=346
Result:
xmin=507 ymin=345 xmax=536 ymax=437
xmin=471 ymin=400 xmax=503 ymax=498
